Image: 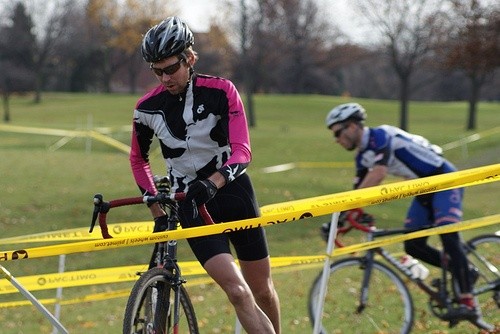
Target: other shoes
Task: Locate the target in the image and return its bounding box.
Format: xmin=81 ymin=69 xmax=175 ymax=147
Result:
xmin=447 ymin=306 xmax=482 ymax=318
xmin=467 ymin=260 xmax=479 ymax=287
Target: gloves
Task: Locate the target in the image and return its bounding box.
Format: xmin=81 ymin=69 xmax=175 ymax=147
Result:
xmin=186 ymin=180 xmax=218 ymax=207
xmin=152 ymin=214 xmax=169 ymax=234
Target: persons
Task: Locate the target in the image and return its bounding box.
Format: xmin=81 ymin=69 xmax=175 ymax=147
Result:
xmin=326 ymin=104 xmax=481 ymax=321
xmin=128 ymin=16 xmax=282 ymax=334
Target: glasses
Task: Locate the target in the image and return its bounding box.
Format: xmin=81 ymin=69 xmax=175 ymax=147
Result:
xmin=335 ymin=127 xmax=348 ymax=137
xmin=150 ymin=58 xmax=184 ymax=76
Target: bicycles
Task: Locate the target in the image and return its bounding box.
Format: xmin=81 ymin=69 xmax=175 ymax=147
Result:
xmin=306 ymin=209 xmax=500 ymax=334
xmin=88 ymin=176 xmax=199 ymax=334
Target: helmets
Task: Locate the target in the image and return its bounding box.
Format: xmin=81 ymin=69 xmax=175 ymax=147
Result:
xmin=141 ymin=16 xmax=194 ymax=62
xmin=327 ymin=103 xmax=366 ymax=129
